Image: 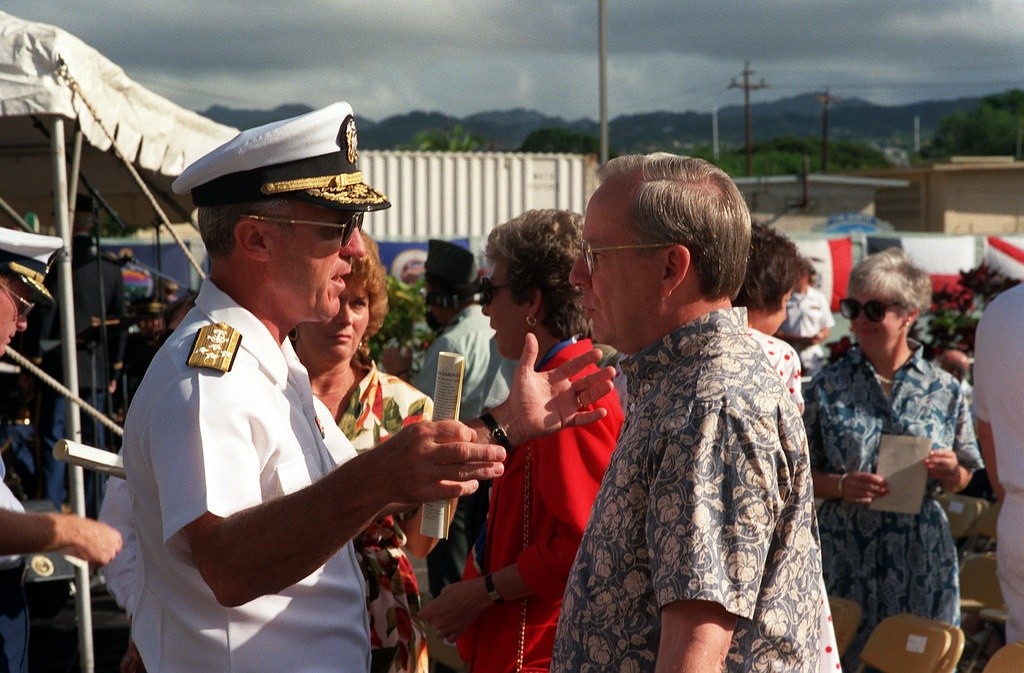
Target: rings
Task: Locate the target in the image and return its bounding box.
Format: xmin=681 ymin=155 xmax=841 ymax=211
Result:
xmin=576 ymin=394 xmax=587 ymax=408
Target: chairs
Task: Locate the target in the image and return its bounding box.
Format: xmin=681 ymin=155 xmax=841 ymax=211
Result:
xmin=823 ymin=495 xmax=1024 ymax=672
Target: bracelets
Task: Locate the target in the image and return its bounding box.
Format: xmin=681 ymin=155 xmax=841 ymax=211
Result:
xmin=480 ymin=412 xmax=511 ymax=451
xmin=837 ymin=473 xmax=849 ymax=498
xmin=485 ymin=574 xmax=503 ymax=606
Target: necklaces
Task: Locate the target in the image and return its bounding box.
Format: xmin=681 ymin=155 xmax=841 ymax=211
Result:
xmin=878 ymin=374 xmax=893 ymax=384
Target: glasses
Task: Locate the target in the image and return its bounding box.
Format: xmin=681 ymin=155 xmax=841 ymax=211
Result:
xmin=840 ymin=298 xmax=896 ymax=322
xmin=0 ymin=283 xmax=35 ymax=320
xmin=580 ymin=240 xmax=698 ymax=277
xmin=480 ymin=277 xmax=509 ymax=305
xmin=240 ymin=211 xmax=363 ymax=249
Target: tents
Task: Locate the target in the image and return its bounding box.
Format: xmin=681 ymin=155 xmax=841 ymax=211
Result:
xmin=0 ymin=7 xmax=247 ymax=673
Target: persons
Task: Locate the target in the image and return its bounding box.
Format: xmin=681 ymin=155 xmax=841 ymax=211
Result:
xmin=418 ymin=208 xmax=625 ymax=673
xmin=731 ymin=217 xmax=809 ymax=415
xmin=939 ymin=350 xmax=996 ymax=501
xmin=967 ymin=282 xmax=1024 ymax=644
xmin=289 ymin=226 xmax=458 ymax=673
xmin=549 ymin=151 xmax=823 ymax=673
xmin=0 ymin=223 xmax=197 ymax=672
xmin=801 ymin=249 xmax=988 ymax=673
xmin=120 ymin=101 xmax=620 ymax=673
xmin=772 ymin=258 xmax=834 ymax=377
xmin=415 ymin=239 xmax=524 ymax=599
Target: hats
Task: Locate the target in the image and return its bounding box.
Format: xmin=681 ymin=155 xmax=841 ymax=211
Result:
xmin=130 ymin=302 xmax=167 ymax=320
xmin=157 ymin=276 xmax=177 ymax=293
xmin=172 ymin=103 xmax=392 ymax=212
xmin=0 ymin=228 xmax=63 ymax=305
xmin=423 ymin=239 xmax=474 ymax=298
xmin=52 ymin=191 xmax=93 ymax=216
